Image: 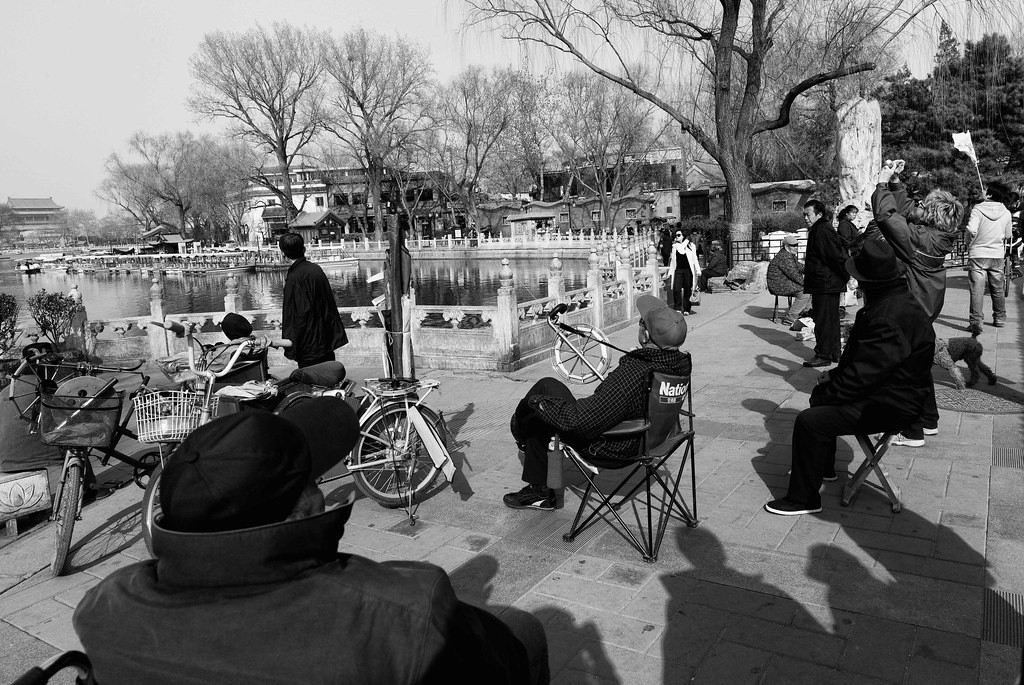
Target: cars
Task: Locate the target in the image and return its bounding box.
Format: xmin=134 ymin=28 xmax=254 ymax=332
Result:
xmin=223 ymin=241 xmax=237 ymax=245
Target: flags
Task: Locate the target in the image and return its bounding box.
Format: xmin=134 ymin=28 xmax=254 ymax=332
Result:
xmin=952 ymin=132 xmax=976 ymax=163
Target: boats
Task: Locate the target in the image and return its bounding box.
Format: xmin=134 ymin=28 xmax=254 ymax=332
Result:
xmin=14 ymin=258 xmax=45 ymax=271
xmin=77 ymin=263 xmax=95 ymax=273
xmin=56 ymin=265 xmax=78 ymax=274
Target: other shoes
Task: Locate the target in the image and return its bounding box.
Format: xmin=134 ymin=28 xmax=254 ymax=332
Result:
xmin=1013 ymin=266 xmax=1023 ymax=278
xmin=783 ymin=319 xmax=792 ymax=326
xmin=676 ymin=311 xmax=689 ymax=315
xmin=967 ymin=322 xmax=983 ymax=332
xmin=993 ymin=318 xmax=1004 ymax=327
xmin=803 ymin=351 xmax=841 ymax=367
xmin=823 ymin=470 xmax=838 ymax=481
xmin=765 ymin=497 xmax=822 ymax=514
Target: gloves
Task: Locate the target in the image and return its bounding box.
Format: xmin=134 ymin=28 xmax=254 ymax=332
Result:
xmin=513 ymin=397 xmax=532 ymax=429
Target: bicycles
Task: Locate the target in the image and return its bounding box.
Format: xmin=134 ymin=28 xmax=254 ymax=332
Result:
xmin=36 ymin=351 xmax=178 ymax=576
xmin=1004 ymin=255 xmax=1018 ymax=297
xmin=132 ymin=320 xmax=463 ymax=559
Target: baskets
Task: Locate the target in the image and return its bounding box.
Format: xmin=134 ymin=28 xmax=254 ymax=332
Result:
xmin=132 ymin=389 xmax=219 ymax=443
xmin=41 ymin=389 xmax=126 ymax=447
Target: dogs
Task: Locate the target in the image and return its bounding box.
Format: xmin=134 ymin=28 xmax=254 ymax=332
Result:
xmin=931 ymin=324 xmax=997 ymax=391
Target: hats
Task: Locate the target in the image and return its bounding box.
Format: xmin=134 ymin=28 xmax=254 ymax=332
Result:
xmin=636 ymin=295 xmax=687 ymax=349
xmin=222 ymin=313 xmax=253 ymax=341
xmin=784 ymin=236 xmax=799 ymax=245
xmin=159 ymin=394 xmax=360 ymax=533
xmin=845 ymin=236 xmax=902 ymax=282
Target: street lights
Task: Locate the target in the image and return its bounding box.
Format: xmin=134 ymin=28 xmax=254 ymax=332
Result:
xmin=240 ymin=224 xmax=248 ymax=242
xmin=716 ymin=193 xmax=725 ymax=220
xmin=568 ymin=198 xmax=572 ymax=228
xmin=428 ymin=212 xmax=438 ymax=233
xmin=324 ymin=219 xmax=332 ymax=243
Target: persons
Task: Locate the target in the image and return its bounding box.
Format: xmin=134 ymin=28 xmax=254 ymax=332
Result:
xmin=355 ymin=236 xmax=359 ymax=242
xmin=767 ymin=236 xmax=812 ymax=325
xmin=836 ymin=204 xmax=861 ymax=242
xmin=206 ymin=313 xmax=262 ymax=364
xmin=627 ymin=223 xmax=634 ymax=235
xmin=870 ymin=160 xmax=964 ymax=446
xmin=766 ymin=240 xmax=936 ymax=515
xmin=965 ymin=181 xmax=1012 ymax=331
xmin=697 ymin=240 xmax=727 ymax=293
xmin=502 ymin=295 xmax=692 ymax=511
xmin=279 ymin=233 xmax=349 ymax=369
xmin=73 ymin=396 xmax=552 ymax=685
xmin=311 ymin=236 xmax=318 ymax=244
xmin=0 ymin=342 xmax=68 ymax=495
xmin=555 ymin=225 xmax=559 ymax=233
xmin=802 ymin=199 xmax=851 ymax=367
xmin=655 ymin=222 xmax=700 ymax=267
xmin=667 ymin=229 xmax=702 ymax=315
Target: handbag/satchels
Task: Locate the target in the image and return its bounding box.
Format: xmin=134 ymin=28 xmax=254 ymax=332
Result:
xmin=689 ymin=274 xmax=701 ymax=305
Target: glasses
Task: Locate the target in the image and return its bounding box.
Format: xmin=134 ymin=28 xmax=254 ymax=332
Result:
xmin=639 ymin=317 xmax=647 ymax=329
xmin=919 ymin=200 xmax=927 ymax=210
xmin=676 ymin=233 xmax=682 ymax=237
xmin=300 ymin=477 xmax=322 ymax=500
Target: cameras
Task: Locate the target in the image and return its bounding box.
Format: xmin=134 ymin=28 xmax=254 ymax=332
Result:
xmin=889 ymin=163 xmax=900 ymax=169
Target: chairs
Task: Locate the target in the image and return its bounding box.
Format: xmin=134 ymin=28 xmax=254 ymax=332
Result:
xmin=562 ymin=372 xmax=700 ymax=563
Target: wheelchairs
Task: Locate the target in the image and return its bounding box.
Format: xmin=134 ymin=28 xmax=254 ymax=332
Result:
xmin=10 ymin=651 xmax=96 ymax=685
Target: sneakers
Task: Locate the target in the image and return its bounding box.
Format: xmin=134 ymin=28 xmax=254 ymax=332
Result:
xmin=923 ymin=427 xmax=938 ymax=435
xmin=503 ymin=484 xmax=557 ymax=510
xmin=510 ymin=413 xmax=522 ymax=443
xmin=878 ymin=432 xmax=925 ymax=447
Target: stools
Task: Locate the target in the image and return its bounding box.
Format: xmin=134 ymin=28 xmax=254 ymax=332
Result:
xmin=773 ymin=294 xmax=794 ymax=324
xmin=842 ymin=429 xmax=902 ymax=514
xmin=0 ymin=468 xmax=52 ymax=536
